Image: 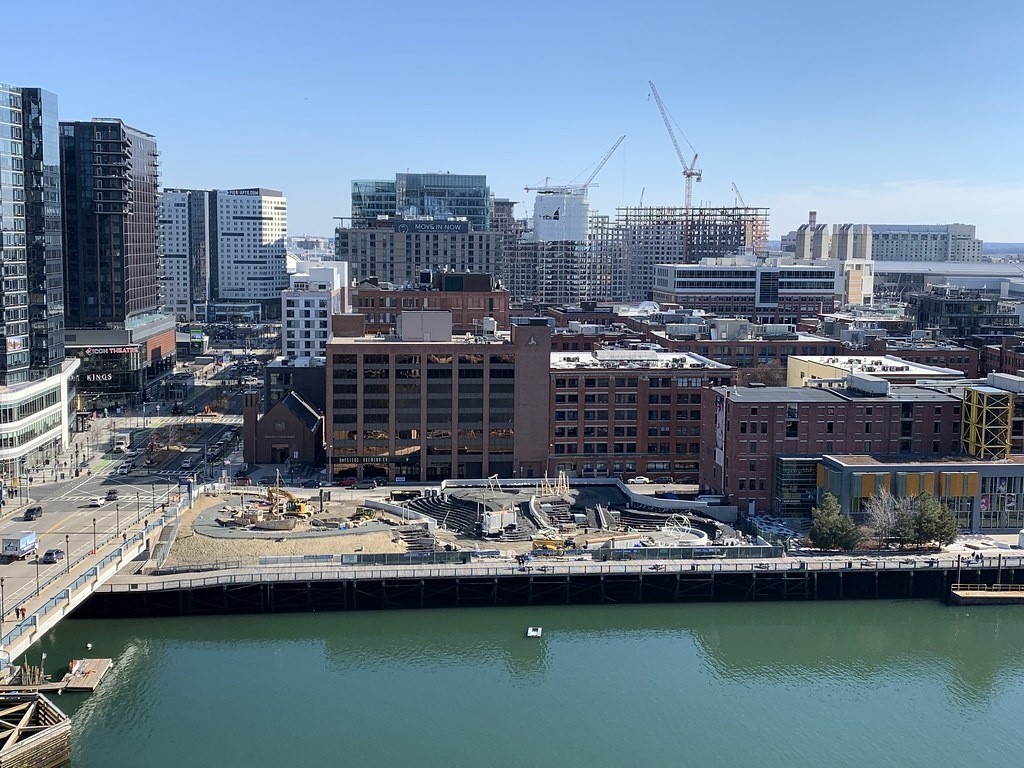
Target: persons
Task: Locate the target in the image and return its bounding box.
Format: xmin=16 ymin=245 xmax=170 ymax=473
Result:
xmin=14 ymin=606 xmax=21 ymax=619
xmin=161 ymin=502 xmax=165 ymax=511
xmin=144 ymin=518 xmax=148 ymax=528
xmin=20 ymin=605 xmax=27 ymax=620
xmin=123 ymin=531 xmax=128 ymax=542
xmin=0 ymin=447 xmax=88 ymax=510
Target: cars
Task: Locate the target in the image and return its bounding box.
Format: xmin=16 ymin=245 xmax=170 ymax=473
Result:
xmin=170 ymin=402 xmax=197 ymax=416
xmin=112 ymin=420 xmax=240 ymax=477
xmin=202 ymin=323 xmax=237 ymax=337
xmin=232 ymin=352 xmax=259 ymax=383
xmin=230 ymin=473 xmax=387 ymax=491
xmin=1 ymin=488 xmax=118 ymax=563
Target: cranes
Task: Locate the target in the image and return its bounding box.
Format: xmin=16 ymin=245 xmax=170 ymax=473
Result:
xmin=523 ymin=134 xmax=628 ymax=194
xmin=647 ymin=79 xmax=703 ymax=207
xmin=729 ymin=182 xmax=747 ymax=209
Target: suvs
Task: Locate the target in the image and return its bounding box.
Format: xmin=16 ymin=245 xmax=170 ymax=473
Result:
xmin=610 ymin=473 xmax=699 ymax=485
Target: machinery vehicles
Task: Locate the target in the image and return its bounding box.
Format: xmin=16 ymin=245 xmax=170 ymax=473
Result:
xmin=266 ymin=486 xmax=313 ymax=519
xmin=531 ymin=538 xmax=564 ymax=555
xmin=354 ymin=507 xmax=386 ymax=523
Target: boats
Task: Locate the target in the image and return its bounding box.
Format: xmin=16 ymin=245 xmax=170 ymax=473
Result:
xmin=527 ymin=626 xmax=542 ymax=638
